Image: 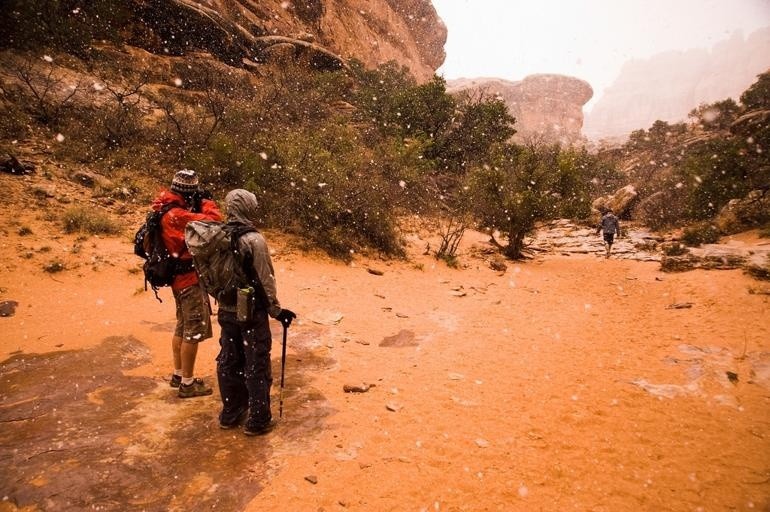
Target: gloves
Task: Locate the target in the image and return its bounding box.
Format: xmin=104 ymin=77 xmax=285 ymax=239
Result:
xmin=276 ymin=309 xmax=296 ymax=327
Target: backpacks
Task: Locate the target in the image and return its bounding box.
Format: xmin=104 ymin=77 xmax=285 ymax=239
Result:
xmin=184 ymin=220 xmax=258 ymax=305
xmin=134 ymin=200 xmax=188 ymax=304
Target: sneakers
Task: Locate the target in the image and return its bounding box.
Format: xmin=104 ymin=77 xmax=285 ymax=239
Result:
xmin=169 ymin=374 xmax=213 ymax=399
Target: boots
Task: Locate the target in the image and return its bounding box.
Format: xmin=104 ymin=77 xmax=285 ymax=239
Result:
xmin=243 ymin=374 xmax=277 ymax=436
xmin=216 ymin=370 xmax=248 ymax=430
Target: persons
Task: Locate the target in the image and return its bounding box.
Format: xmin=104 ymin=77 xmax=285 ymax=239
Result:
xmin=152 ymin=170 xmax=224 ymax=398
xmin=595 ymin=210 xmax=621 ymax=259
xmin=216 ymin=188 xmax=296 ymax=436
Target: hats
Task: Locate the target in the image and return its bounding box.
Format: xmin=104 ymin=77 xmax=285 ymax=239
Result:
xmin=171 ymin=169 xmax=199 ymax=193
